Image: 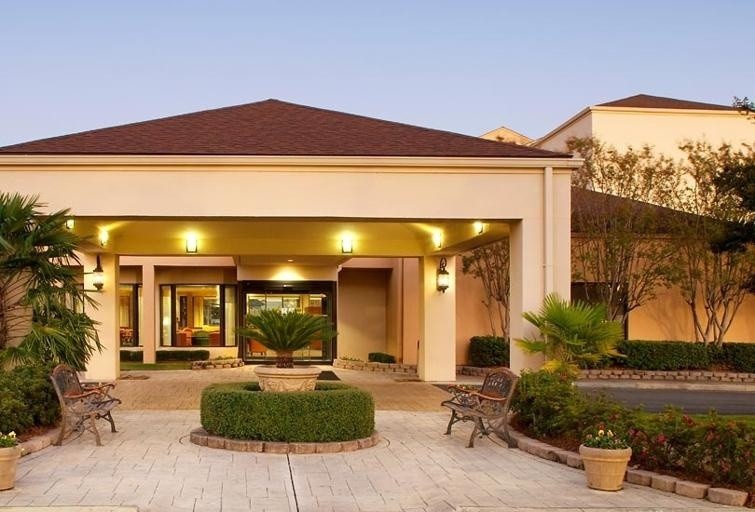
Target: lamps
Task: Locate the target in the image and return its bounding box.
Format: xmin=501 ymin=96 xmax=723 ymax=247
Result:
xmin=436 ymin=256 xmax=451 ymax=293
xmin=92 ymin=252 xmax=104 ymax=291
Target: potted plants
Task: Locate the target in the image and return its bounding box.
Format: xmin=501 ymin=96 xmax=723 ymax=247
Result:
xmin=231 ymin=307 xmax=340 ymax=393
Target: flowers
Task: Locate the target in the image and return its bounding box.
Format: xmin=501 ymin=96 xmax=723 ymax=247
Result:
xmin=0 ymin=429 xmax=21 ymax=448
xmin=584 ymin=428 xmax=627 ymax=447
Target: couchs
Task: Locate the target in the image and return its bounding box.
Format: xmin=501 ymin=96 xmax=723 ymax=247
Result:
xmin=177 ymin=328 xmax=221 ymax=347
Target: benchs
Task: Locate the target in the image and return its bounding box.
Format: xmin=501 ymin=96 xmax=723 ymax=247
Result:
xmin=441 ymin=368 xmax=519 ymax=447
xmin=50 ymin=363 xmax=122 ymax=447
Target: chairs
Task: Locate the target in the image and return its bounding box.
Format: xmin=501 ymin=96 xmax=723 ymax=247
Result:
xmin=248 ymin=337 xmax=268 ymax=356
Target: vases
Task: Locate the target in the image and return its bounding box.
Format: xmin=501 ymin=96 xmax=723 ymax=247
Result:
xmin=578 ymin=443 xmax=632 ymax=491
xmin=0 ymin=445 xmax=20 ymax=489
